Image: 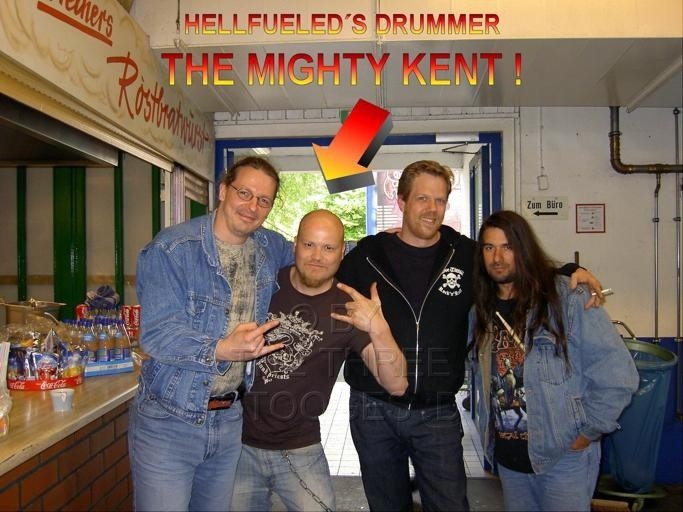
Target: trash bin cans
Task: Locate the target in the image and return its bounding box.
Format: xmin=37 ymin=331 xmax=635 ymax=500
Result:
xmin=0 ymin=298 xmax=66 ymax=325
xmin=596 ymin=320 xmax=682 ymax=511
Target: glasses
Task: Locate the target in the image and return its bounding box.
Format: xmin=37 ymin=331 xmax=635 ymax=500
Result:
xmin=227 ymin=181 xmax=273 ymax=208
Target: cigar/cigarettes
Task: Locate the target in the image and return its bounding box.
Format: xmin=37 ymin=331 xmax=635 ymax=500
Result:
xmin=591 ymin=287 xmax=611 ymax=297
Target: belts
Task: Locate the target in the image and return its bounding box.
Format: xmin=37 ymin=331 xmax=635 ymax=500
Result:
xmin=207 ymin=386 xmax=244 ymax=411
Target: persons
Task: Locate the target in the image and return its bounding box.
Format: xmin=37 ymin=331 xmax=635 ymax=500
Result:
xmin=126 ymin=156 xmax=402 ymax=512
xmin=334 ymin=159 xmax=606 ymax=512
xmin=464 ymin=208 xmax=642 ymax=512
xmin=230 ymin=209 xmax=410 ymax=512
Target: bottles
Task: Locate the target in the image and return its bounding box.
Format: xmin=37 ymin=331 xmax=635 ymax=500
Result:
xmin=64 ymin=303 xmax=130 ymax=363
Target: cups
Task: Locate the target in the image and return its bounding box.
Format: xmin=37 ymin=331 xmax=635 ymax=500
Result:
xmin=50 ymin=388 xmax=75 ymax=413
